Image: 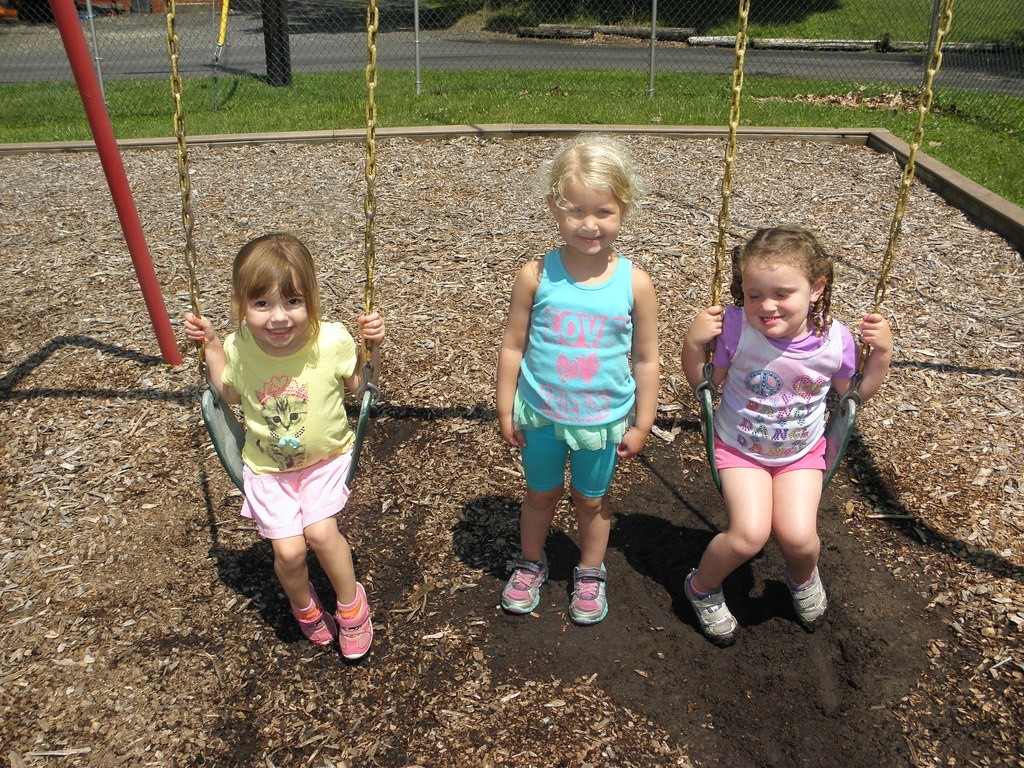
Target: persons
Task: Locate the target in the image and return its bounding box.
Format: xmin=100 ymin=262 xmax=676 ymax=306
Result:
xmin=184 ymin=231 xmax=387 ymax=660
xmin=682 ymin=224 xmax=893 ymax=648
xmin=494 ymin=140 xmax=660 ymax=626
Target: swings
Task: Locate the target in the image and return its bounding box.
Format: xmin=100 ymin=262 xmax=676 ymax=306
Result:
xmin=164 ymin=0 xmax=383 ymax=496
xmin=694 ymin=0 xmax=958 ymax=500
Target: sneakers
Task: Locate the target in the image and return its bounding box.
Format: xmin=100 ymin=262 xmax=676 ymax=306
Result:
xmin=290 ymin=581 xmax=337 ymax=645
xmin=684 ymin=570 xmax=741 ymax=645
xmin=569 ymin=561 xmax=609 ymax=624
xmin=332 ymin=581 xmax=373 ymax=659
xmin=783 ymin=563 xmax=829 ymax=630
xmin=502 ymin=550 xmax=549 ymax=613
